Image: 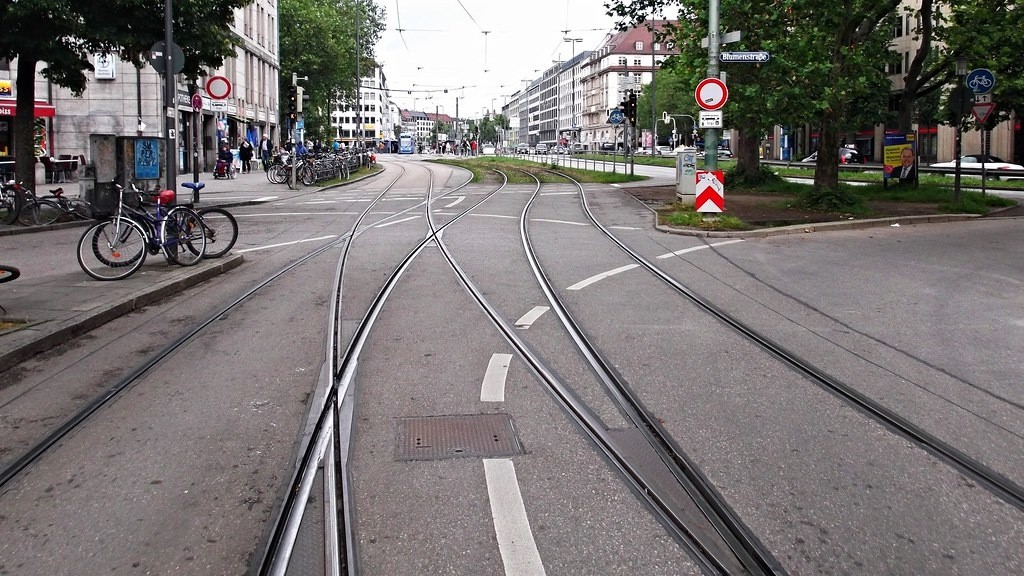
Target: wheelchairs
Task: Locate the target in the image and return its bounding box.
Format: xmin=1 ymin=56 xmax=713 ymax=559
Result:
xmin=213 ymin=159 xmax=237 ymax=179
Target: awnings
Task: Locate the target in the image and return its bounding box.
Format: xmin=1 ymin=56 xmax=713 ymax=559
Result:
xmin=0 ymin=98 xmax=56 ymax=118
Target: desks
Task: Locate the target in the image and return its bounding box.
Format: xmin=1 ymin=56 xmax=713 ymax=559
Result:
xmin=52 ymin=160 xmax=79 ymax=183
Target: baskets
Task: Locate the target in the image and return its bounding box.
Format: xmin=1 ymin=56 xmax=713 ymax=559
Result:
xmin=88 ymin=187 xmax=116 ymax=219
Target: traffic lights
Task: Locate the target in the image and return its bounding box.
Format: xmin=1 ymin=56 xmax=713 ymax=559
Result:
xmin=287 ymin=85 xmax=297 ymax=111
xmin=620 ymin=101 xmax=628 ymax=116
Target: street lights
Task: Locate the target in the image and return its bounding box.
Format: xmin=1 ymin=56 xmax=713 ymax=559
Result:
xmin=521 ymin=80 xmax=533 ymax=143
xmin=500 ymin=95 xmax=510 ymax=141
xmin=563 ymin=37 xmax=583 ymax=124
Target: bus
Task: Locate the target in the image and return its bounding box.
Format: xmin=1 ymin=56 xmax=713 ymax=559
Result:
xmin=397 ymin=133 xmax=414 ymax=154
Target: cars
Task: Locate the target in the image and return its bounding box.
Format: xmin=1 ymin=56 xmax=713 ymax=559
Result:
xmin=535 ymin=144 xmax=548 ymax=155
xmin=928 ymin=155 xmax=1024 ymax=183
xmin=481 ymin=144 xmax=496 ymax=152
xmin=515 ymin=143 xmax=529 ymax=154
xmin=600 ymin=141 xmax=733 ymax=159
xmin=801 ymin=147 xmax=866 ymax=174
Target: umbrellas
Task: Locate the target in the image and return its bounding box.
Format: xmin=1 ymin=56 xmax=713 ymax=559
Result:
xmin=443 ymin=140 xmax=454 ymax=143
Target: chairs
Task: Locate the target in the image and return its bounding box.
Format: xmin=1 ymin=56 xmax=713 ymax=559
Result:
xmin=249 ymin=151 xmax=261 ymax=172
xmin=39 ymin=154 xmax=93 ymax=183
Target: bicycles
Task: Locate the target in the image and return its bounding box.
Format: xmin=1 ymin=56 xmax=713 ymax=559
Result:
xmin=17 ymin=181 xmax=100 ymax=227
xmin=0 ymin=265 xmax=21 ymax=284
xmin=76 ymin=176 xmax=207 ymax=281
xmin=0 ymin=171 xmax=22 ymax=226
xmin=266 ymin=147 xmax=376 ymax=186
xmin=91 ymin=177 xmax=238 ymax=268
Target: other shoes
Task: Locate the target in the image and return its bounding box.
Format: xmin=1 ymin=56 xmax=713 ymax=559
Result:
xmin=248 ymin=170 xmax=251 ymax=174
xmin=243 ymin=171 xmax=247 ymax=174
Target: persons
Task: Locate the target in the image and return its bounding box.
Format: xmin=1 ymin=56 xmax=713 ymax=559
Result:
xmin=379 ymin=141 xmax=384 ymax=153
xmin=283 ymin=139 xmax=321 ymax=156
xmin=259 ymin=134 xmax=273 ymax=172
xmin=890 ymin=147 xmax=915 ymax=178
xmin=340 ymin=141 xmax=345 ymax=152
xmin=239 ymin=137 xmax=253 ymax=173
xmin=218 ymin=145 xmax=234 ymax=172
xmin=418 ymin=138 xmax=477 ymax=156
xmin=333 ymin=140 xmax=339 ymax=154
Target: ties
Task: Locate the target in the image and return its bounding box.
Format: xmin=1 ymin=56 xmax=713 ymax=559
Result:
xmin=902 ymin=168 xmax=908 ymax=177
